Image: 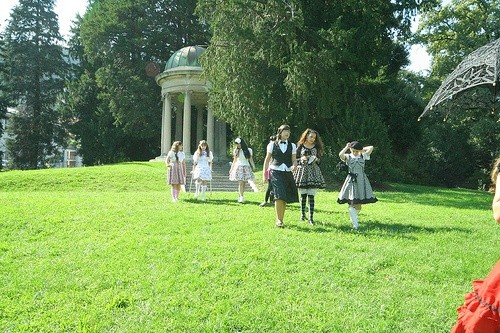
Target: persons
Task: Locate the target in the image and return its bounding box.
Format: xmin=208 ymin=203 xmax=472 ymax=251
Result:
xmin=166 ymin=141 xmax=186 ymax=202
xmin=229 ymin=138 xmax=255 ymax=203
xmin=292 ymin=129 xmax=326 ymax=225
xmin=190 ymin=140 xmax=213 ymax=201
xmin=337 ymin=141 xmax=378 ymax=229
xmin=259 ymin=125 xmax=299 ymax=226
xmin=453 ymin=155 xmax=500 ymax=333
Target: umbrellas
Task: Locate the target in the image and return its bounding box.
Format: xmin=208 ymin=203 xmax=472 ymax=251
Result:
xmin=417 ymin=38 xmax=500 ymax=121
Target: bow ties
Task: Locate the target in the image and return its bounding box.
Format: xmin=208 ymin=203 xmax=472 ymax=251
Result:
xmin=280 ymin=141 xmax=286 ymax=144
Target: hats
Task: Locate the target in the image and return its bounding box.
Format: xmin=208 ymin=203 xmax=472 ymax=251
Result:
xmin=350 ymin=141 xmax=363 ymax=150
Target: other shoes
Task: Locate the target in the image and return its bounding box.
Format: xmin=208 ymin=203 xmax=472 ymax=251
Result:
xmin=308 ymin=220 xmax=315 ymax=225
xmin=238 ymin=196 xmax=244 ymax=203
xmin=259 ymin=202 xmax=268 ymax=207
xmin=275 ymin=221 xmax=284 ymax=227
xmin=301 ymin=216 xmax=306 ymax=220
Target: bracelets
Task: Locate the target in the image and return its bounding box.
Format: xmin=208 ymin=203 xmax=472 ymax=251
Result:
xmin=235 ymin=154 xmax=238 ymax=157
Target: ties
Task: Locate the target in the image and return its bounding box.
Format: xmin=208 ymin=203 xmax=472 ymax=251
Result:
xmin=175 ymin=152 xmax=178 ymax=161
